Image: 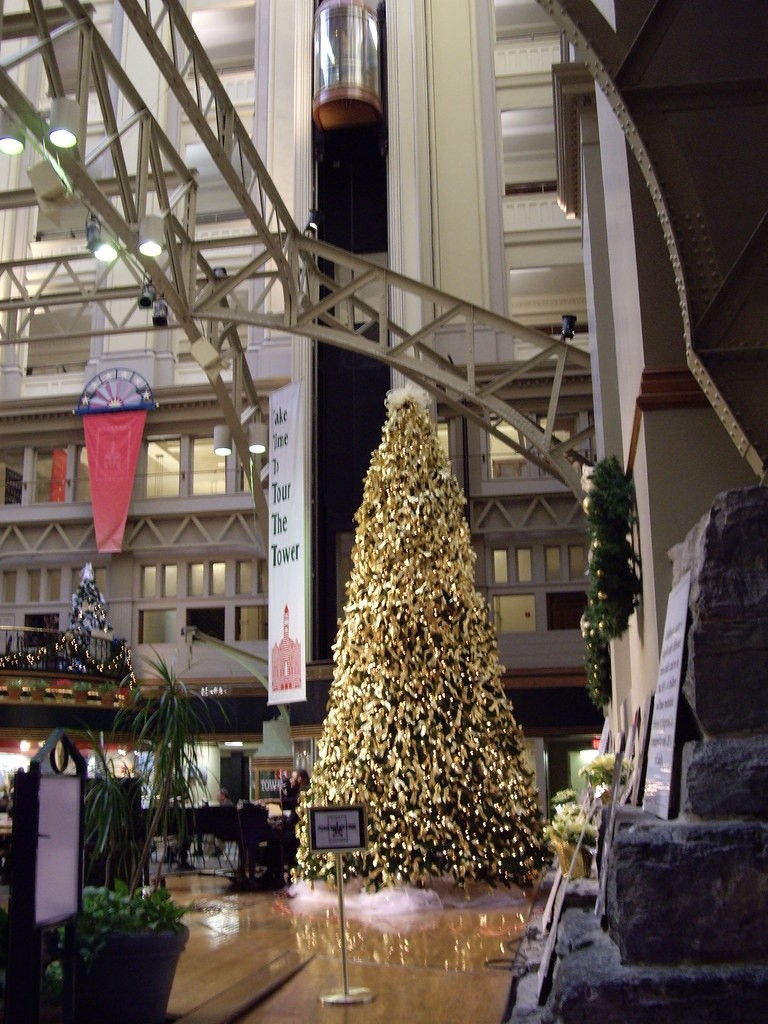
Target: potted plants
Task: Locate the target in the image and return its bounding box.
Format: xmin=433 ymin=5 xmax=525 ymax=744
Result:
xmin=71 ymin=682 xmax=92 ymax=704
xmin=98 ymin=680 xmax=119 ymax=705
xmin=125 ymin=687 xmax=142 ymax=706
xmin=25 ymin=679 xmax=50 ymax=701
xmin=4 ymin=677 xmax=24 ymax=700
xmin=53 ymin=643 xmax=230 ymax=1024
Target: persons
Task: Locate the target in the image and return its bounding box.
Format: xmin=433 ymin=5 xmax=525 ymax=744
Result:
xmin=161 ymin=837 xmax=195 ymax=871
xmin=254 ymin=768 xmax=308 ymax=827
xmin=211 ymin=789 xmax=234 ymax=856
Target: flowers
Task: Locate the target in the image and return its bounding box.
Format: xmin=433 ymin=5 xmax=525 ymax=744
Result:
xmin=577 ymin=751 xmax=634 ymax=797
xmin=553 ymin=801 xmax=592 ymax=830
xmin=547 ymin=786 xmax=578 ymax=810
xmin=539 ymin=819 xmax=598 ymax=854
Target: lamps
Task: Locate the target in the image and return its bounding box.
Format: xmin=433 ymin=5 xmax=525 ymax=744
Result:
xmin=152 ymin=302 xmax=168 ymax=327
xmin=249 ymin=422 xmax=268 ymax=454
xmin=213 ymin=424 xmax=232 ymax=456
xmin=561 ymin=315 xmax=577 ymax=339
xmin=139 ymin=215 xmax=164 ymax=257
xmin=93 ymin=227 xmax=119 ymax=263
xmin=0 ymin=110 xmax=25 ymax=156
xmin=308 ymin=209 xmax=322 ymax=230
xmin=137 ymin=288 xmax=156 ymax=309
xmin=48 ymin=97 xmax=81 ymax=150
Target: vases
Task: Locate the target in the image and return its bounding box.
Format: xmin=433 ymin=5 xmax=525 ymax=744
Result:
xmin=555 ymin=846 xmax=594 ymax=880
xmin=601 ymin=791 xmax=611 ymax=804
